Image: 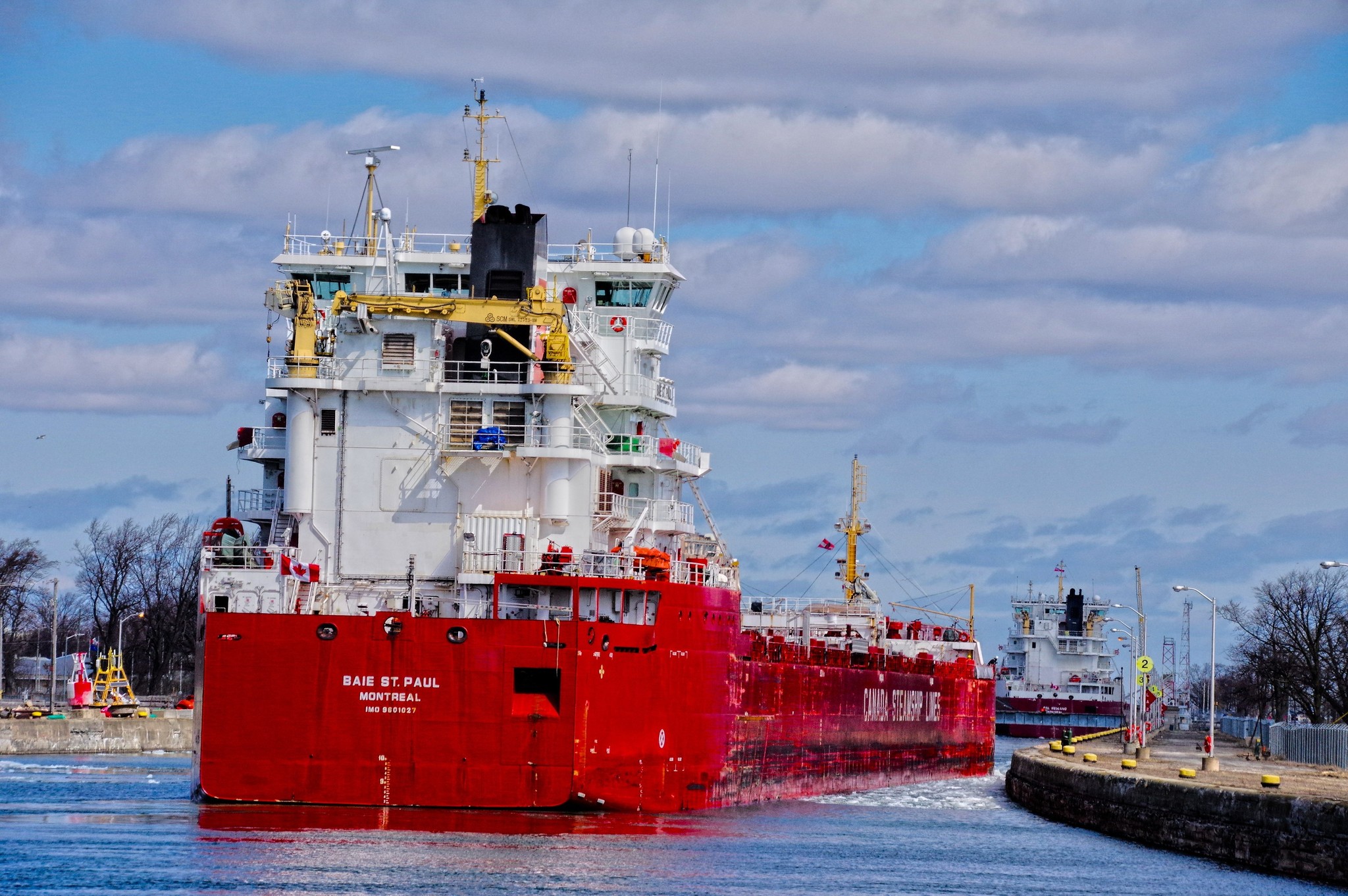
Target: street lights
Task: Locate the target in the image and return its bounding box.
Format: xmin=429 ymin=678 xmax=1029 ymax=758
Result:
xmin=1102 ymin=614 xmax=1161 ymax=743
xmin=1171 ymin=586 xmax=1215 ymax=757
xmin=115 ymin=612 xmax=146 ymax=704
xmin=1110 ymin=602 xmax=1147 ymax=751
xmin=63 ymin=634 xmax=86 ymax=703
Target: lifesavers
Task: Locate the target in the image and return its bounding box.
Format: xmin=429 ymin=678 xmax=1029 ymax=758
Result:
xmin=1125 ymin=729 xmax=1130 ymax=741
xmin=1139 ymin=731 xmax=1143 ymax=745
xmin=959 ymin=632 xmax=969 ymax=643
xmin=1132 ymin=724 xmax=1134 ymax=733
xmin=1204 ymin=736 xmax=1211 ymax=753
xmin=1145 ymin=722 xmax=1151 ymax=731
xmin=1136 ymin=726 xmax=1140 ymax=731
xmin=610 ymin=315 xmax=627 ymax=332
xmin=313 ymin=310 xmax=325 ymax=324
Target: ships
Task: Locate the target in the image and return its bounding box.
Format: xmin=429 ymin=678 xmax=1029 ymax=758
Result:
xmin=194 ymin=77 xmax=999 ymax=836
xmin=993 ymin=559 xmax=1127 ymax=740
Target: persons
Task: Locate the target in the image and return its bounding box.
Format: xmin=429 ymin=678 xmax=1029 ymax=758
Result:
xmin=988 ymin=656 xmax=998 ymax=666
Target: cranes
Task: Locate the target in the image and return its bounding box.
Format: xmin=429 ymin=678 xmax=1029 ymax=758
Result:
xmin=1134 ymin=561 xmax=1147 ymax=678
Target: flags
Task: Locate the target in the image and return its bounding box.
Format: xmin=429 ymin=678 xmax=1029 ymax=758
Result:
xmin=1050 ymin=684 xmax=1059 ymax=690
xmin=1115 ymin=650 xmax=1119 ymax=655
xmin=91 ymin=645 xmax=97 ymax=651
xmin=818 ymin=539 xmax=835 ymax=550
xmin=90 ymin=639 xmax=99 ymax=645
xmin=280 ymin=554 xmax=320 ymax=583
xmin=1055 ymin=568 xmax=1064 ymax=572
xmin=999 ymin=646 xmax=1003 ymax=650
